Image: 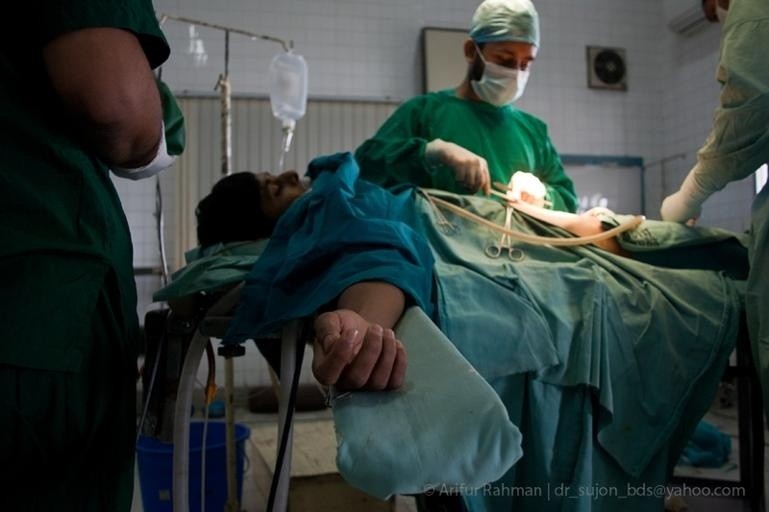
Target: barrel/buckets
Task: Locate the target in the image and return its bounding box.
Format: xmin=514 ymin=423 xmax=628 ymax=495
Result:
xmin=137 ymin=419 xmax=249 ymax=512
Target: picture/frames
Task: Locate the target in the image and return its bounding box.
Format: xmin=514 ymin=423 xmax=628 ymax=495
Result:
xmin=422 ymin=26 xmax=473 ymax=95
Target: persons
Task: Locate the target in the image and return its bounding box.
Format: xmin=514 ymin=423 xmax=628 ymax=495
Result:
xmin=661 ymin=1 xmax=769 ymax=512
xmin=193 ymin=169 xmax=749 ymax=393
xmin=354 ymin=0 xmax=580 ymax=214
xmin=0 ymin=0 xmax=176 ymax=512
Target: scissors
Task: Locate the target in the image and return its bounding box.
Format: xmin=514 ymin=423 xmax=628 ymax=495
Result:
xmin=485 ymin=206 xmax=523 ymax=262
xmin=418 ymin=187 xmax=461 ymax=234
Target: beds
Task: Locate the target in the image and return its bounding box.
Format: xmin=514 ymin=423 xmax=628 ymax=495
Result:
xmin=139 ymin=305 xmax=767 ymax=512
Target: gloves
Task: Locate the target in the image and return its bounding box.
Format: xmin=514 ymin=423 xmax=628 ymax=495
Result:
xmin=426 ymin=138 xmax=491 ymax=196
xmin=507 ymin=170 xmax=546 ymax=207
xmin=660 ymin=165 xmax=712 ymax=222
xmin=114 ymin=122 xmax=178 ymax=178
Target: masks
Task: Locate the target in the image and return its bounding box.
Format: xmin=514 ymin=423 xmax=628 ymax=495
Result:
xmin=468 ymin=44 xmax=529 ymax=106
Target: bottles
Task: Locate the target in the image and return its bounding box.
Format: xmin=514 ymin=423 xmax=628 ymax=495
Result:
xmin=270 ymin=55 xmax=309 ymax=136
